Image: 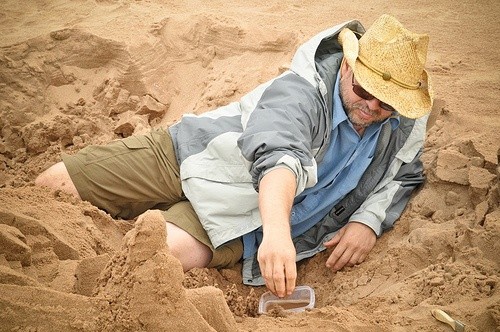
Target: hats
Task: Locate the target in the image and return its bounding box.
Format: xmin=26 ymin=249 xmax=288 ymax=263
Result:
xmin=337 ymin=14 xmax=434 ymax=120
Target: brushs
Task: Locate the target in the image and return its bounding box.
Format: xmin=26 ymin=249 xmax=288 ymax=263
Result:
xmin=430 ymin=308 xmax=470 ymax=332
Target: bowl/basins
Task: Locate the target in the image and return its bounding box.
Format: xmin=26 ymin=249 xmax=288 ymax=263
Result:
xmin=258 ymin=286 xmax=316 ymax=317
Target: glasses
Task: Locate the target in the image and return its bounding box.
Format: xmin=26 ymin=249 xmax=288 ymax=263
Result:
xmin=351 ymin=73 xmax=395 ymax=112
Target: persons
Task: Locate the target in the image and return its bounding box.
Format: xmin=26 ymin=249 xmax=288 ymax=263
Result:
xmin=35 ymin=13 xmax=434 ymax=298
